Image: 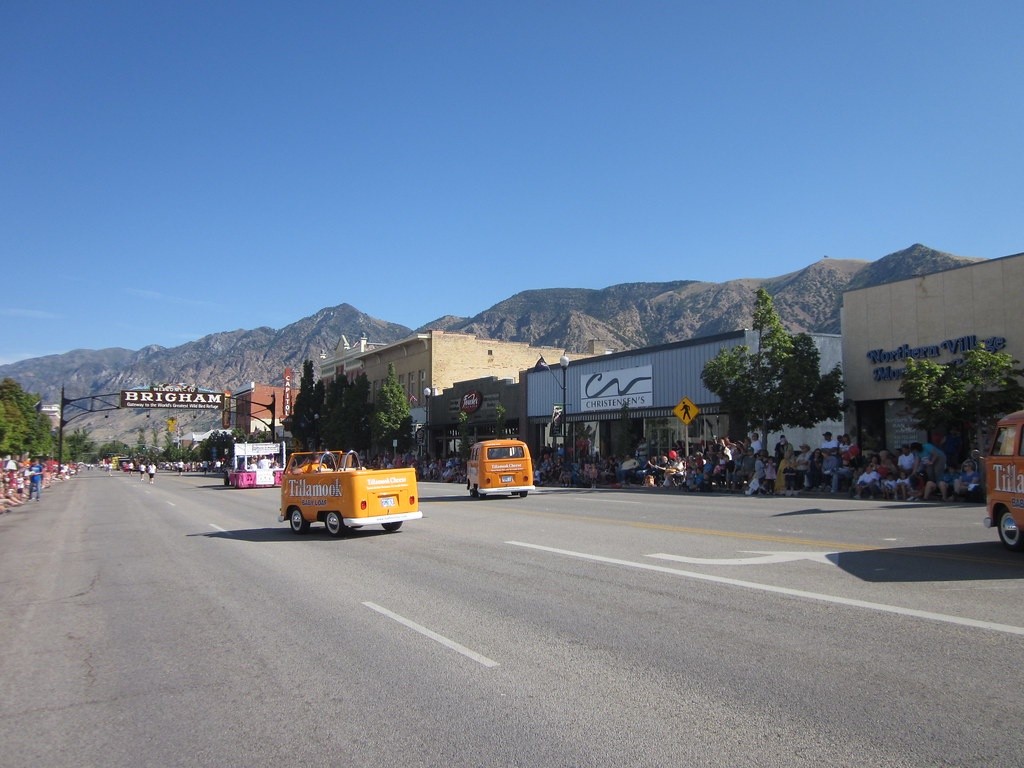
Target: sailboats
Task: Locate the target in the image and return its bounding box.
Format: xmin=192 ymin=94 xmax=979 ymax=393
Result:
xmin=560 ymin=356 xmax=569 ymax=458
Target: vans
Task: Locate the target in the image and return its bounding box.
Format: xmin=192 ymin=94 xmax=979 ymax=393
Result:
xmin=278 ymin=450 xmax=423 ymax=537
xmin=467 ymin=439 xmax=535 ymax=499
xmin=982 ymin=409 xmax=1024 ymax=552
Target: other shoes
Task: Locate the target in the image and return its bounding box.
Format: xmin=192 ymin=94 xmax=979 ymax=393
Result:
xmin=946 ymin=497 xmax=955 ymax=502
xmin=908 ymin=497 xmax=918 ymax=501
xmin=150 ymin=481 xmax=153 ymax=485
xmin=919 ymin=496 xmax=928 ymax=501
xmin=140 ymin=479 xmax=144 ymax=482
xmin=37 ymin=499 xmax=39 ymax=503
xmin=855 ymin=494 xmax=861 ymax=499
xmin=28 ymin=497 xmax=35 ymax=501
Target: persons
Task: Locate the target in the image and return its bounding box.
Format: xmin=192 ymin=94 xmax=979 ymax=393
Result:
xmin=129 ymin=462 xmax=134 ymax=476
xmin=292 ymin=452 xmax=327 ymax=475
xmin=258 ymin=455 xmax=271 ymax=469
xmin=186 ymin=462 xmax=190 ymax=472
xmin=87 ymin=463 xmax=93 ymax=470
xmin=0 ymin=457 xmax=80 ymax=514
xmin=216 ymin=460 xmax=221 ymax=473
xmin=148 ymin=462 xmax=156 ymax=484
xmin=249 ymin=458 xmax=257 ymax=469
xmin=203 ymin=461 xmax=208 ymax=474
xmin=177 ymin=459 xmax=184 ymax=477
xmin=139 ymin=463 xmax=146 ymax=481
xmin=108 ymin=462 xmax=113 ymax=477
xmin=533 ymin=431 xmax=986 ymax=502
xmin=363 ymin=451 xmax=467 ymax=483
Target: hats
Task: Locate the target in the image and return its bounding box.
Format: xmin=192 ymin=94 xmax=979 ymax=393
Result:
xmin=822 ymin=432 xmax=833 ymax=437
xmin=748 ymin=447 xmax=754 ymax=453
xmin=254 ymin=455 xmax=258 ymax=458
xmin=799 ymin=442 xmax=810 ymax=450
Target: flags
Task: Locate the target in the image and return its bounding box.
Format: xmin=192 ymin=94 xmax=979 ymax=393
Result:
xmin=533 ymin=357 xmax=549 ymax=373
xmin=408 ymin=392 xmax=417 ymax=402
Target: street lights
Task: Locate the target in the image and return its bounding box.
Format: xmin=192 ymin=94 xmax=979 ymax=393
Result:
xmin=424 ymin=387 xmax=431 ymax=458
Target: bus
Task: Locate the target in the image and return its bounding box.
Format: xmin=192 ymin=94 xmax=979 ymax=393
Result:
xmin=224 ymin=441 xmax=286 ymax=489
